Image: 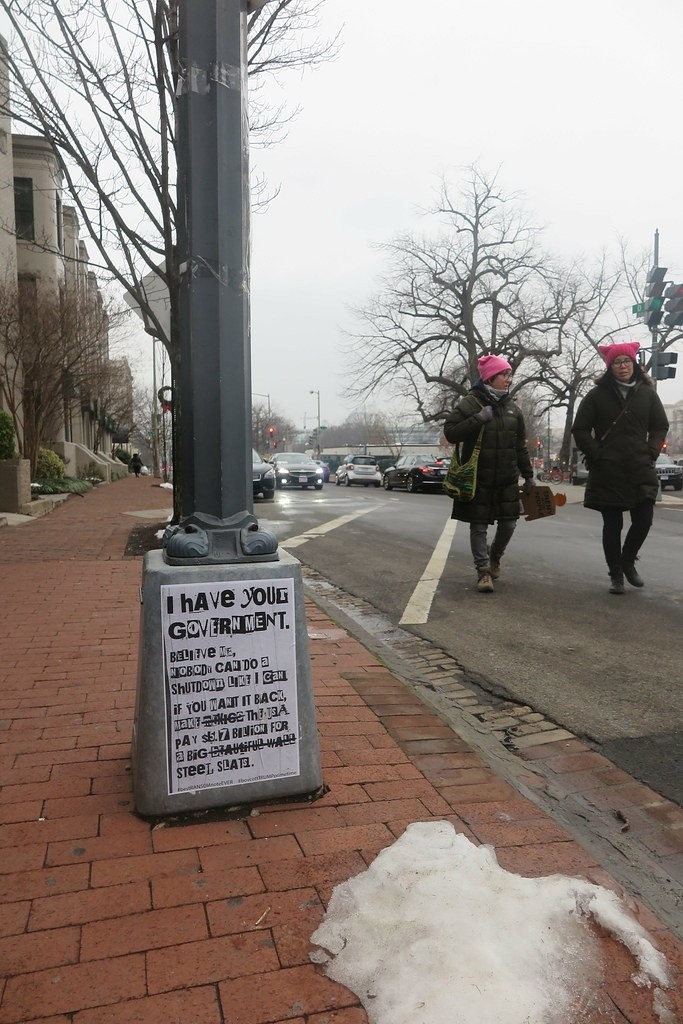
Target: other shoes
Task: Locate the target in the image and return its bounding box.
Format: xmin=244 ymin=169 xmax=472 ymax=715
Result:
xmin=621 ymin=555 xmax=645 ymax=587
xmin=608 ymin=572 xmax=624 ymax=593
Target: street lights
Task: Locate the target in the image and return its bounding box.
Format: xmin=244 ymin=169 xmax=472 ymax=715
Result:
xmin=311 ymin=391 xmax=320 ymax=447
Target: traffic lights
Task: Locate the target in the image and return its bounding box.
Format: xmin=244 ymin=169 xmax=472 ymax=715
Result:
xmin=270 ymin=428 xmax=274 ymax=437
xmin=663 ymin=444 xmax=666 ymax=453
xmin=645 ymin=267 xmax=668 ymax=326
xmin=538 ymin=442 xmax=540 ymax=451
xmin=664 ymin=284 xmax=683 ymax=326
xmin=313 ymin=428 xmax=317 ymax=439
xmin=309 ymin=436 xmax=312 ymax=445
xmin=657 ymin=351 xmax=677 ymax=380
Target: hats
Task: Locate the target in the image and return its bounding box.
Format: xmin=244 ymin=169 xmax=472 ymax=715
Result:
xmin=478 ymin=355 xmax=512 ymax=381
xmin=600 ymin=342 xmax=640 ymax=367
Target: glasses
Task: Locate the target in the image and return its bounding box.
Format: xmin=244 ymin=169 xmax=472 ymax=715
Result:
xmin=496 ymin=373 xmax=513 ymax=380
xmin=612 ymin=357 xmax=634 ymax=367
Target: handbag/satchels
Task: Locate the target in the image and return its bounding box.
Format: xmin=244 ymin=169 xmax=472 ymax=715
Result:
xmin=442 ymin=449 xmax=478 ymax=501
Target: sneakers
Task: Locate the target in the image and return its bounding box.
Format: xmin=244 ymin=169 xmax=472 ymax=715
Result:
xmin=489 ymin=554 xmax=500 ymax=578
xmin=478 ymin=573 xmax=494 ymax=592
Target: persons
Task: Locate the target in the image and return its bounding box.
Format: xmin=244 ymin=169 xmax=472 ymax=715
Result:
xmin=445 ymin=355 xmax=536 ymax=591
xmin=131 ymin=454 xmax=144 ymax=478
xmin=572 ymin=342 xmax=669 ymax=593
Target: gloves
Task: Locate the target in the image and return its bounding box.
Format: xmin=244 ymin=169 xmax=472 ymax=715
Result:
xmin=479 ymin=405 xmax=492 ymax=421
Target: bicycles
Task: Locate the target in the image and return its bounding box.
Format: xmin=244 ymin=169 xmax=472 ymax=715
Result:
xmin=537 ymin=467 xmax=564 ymax=485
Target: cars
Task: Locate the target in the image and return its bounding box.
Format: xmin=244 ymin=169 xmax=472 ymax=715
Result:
xmin=335 ymin=454 xmax=382 ymax=487
xmin=383 ymin=454 xmax=450 ymax=493
xmin=252 ymin=448 xmax=276 ymax=498
xmin=654 ymin=453 xmax=683 ymax=490
xmin=268 ymin=452 xmax=324 ymax=490
xmin=313 ymin=460 xmax=330 ymax=483
